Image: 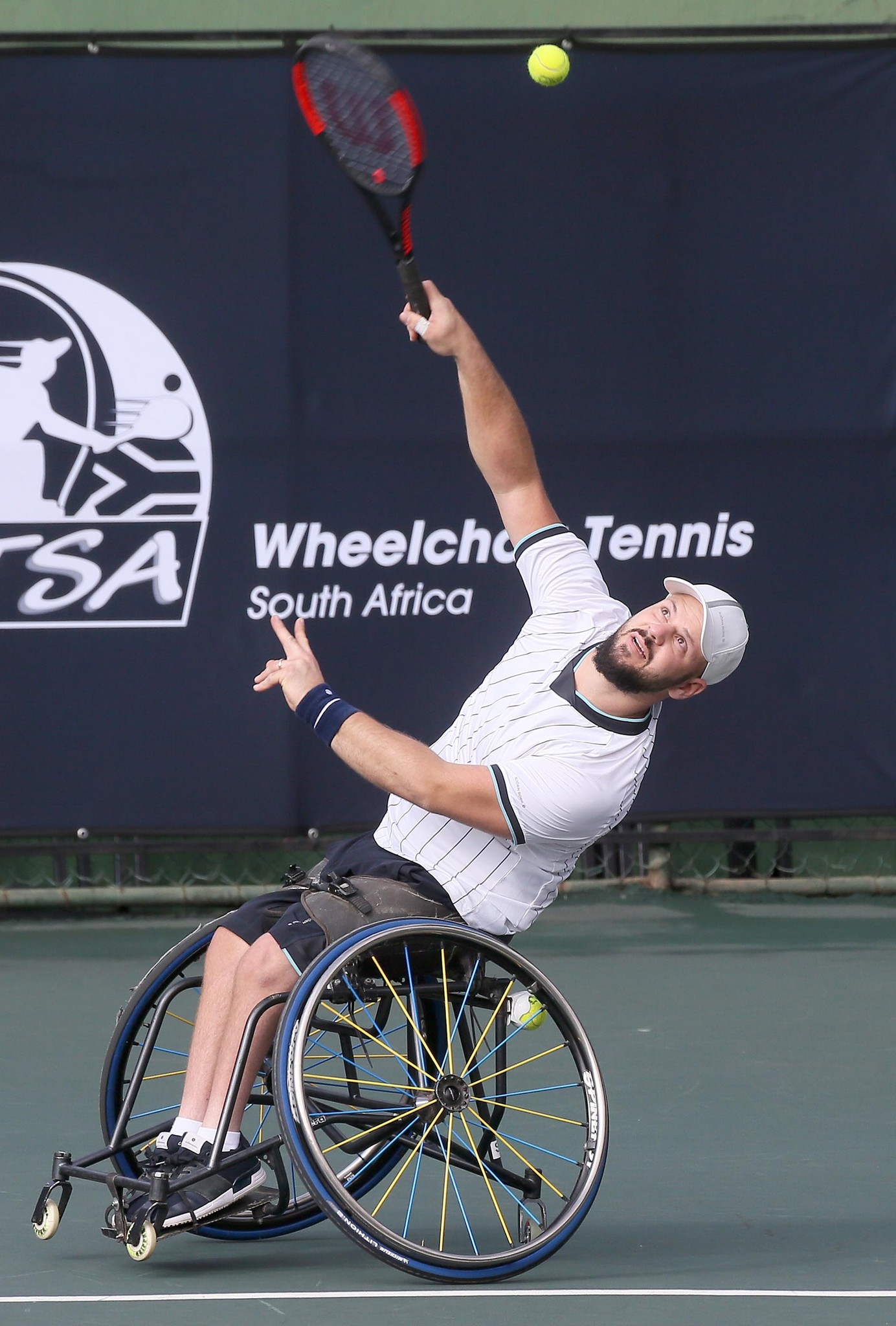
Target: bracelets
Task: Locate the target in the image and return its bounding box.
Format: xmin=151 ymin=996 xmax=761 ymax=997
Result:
xmin=293 ymin=683 xmax=362 ymax=747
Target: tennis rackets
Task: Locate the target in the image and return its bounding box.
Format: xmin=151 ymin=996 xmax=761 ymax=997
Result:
xmin=291 ymin=35 xmax=430 ymax=319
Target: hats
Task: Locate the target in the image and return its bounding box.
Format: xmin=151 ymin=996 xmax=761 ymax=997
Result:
xmin=665 ymin=574 xmax=750 ymax=686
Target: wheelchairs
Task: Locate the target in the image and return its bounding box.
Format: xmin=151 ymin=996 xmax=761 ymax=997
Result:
xmin=26 ymin=893 xmax=610 ymax=1284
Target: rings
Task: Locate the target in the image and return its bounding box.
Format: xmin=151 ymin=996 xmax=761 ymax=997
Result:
xmin=278 ymin=658 xmax=284 ymax=669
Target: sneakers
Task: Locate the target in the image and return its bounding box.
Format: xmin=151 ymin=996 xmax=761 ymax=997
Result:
xmin=133 ymin=1131 xmax=266 ymax=1228
xmin=105 ymin=1131 xmax=182 ymax=1226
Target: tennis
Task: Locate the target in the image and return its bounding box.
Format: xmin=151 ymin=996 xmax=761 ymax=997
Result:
xmin=527 ymin=43 xmax=571 ymax=88
xmin=519 ymin=996 xmax=547 ymax=1030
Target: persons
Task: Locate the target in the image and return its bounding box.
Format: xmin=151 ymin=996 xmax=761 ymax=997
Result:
xmin=103 ymin=278 xmax=749 ymax=1229
